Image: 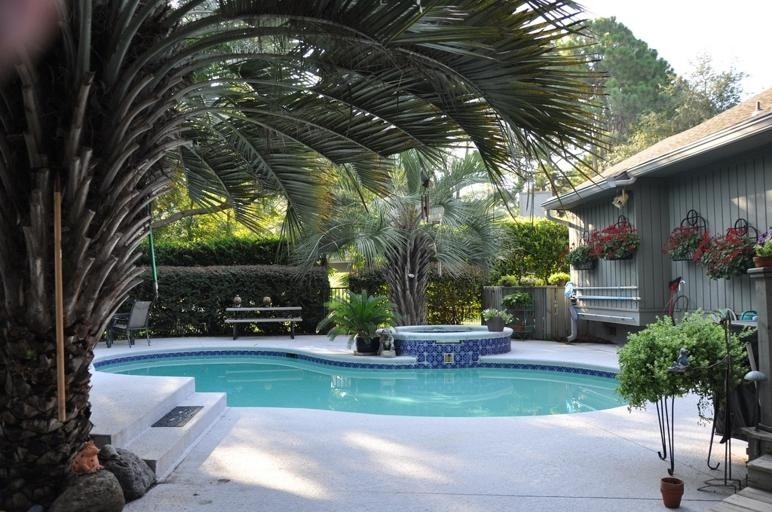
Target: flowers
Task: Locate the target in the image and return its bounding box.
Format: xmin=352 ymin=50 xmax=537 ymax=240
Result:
xmin=559 ymin=221 xmax=640 ymax=271
xmin=660 ymin=224 xmax=772 ymax=281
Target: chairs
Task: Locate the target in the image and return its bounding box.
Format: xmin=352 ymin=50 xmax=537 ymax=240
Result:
xmin=105 ymin=299 xmax=153 ymax=348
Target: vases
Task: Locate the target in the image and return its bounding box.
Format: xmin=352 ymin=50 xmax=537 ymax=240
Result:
xmin=660 ymin=477 xmax=684 ymax=508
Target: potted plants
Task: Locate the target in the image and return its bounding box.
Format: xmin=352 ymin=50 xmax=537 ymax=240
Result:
xmin=316 ymin=288 xmax=404 ymax=356
xmin=479 ymin=269 xmax=572 ymax=332
